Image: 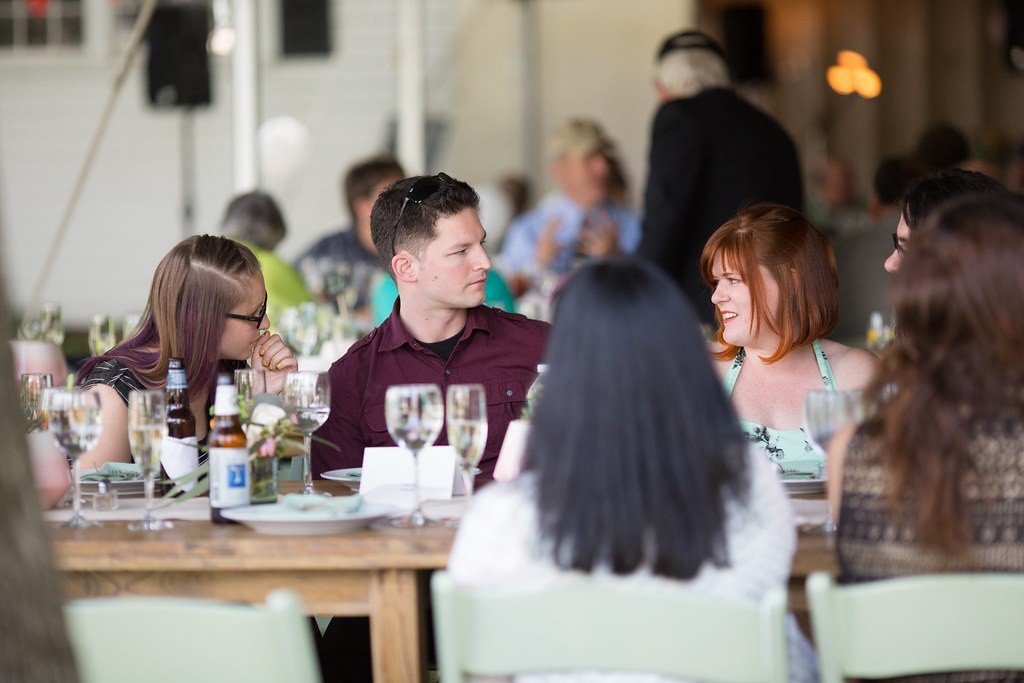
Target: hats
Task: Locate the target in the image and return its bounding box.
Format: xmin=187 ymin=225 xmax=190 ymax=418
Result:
xmin=659 ymin=30 xmax=729 ymax=62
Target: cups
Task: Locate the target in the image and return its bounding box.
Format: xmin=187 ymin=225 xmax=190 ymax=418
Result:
xmin=234 ymin=369 xmax=266 ymax=401
xmin=804 ymin=390 xmax=855 ymax=458
xmin=19 ymin=372 xmax=53 ymax=433
xmin=287 ymin=301 xmax=359 ymax=365
xmin=18 ymin=305 xmax=142 ymax=359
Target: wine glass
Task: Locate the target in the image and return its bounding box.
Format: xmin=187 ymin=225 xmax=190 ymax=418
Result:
xmin=48 ymin=388 xmax=101 ymax=516
xmin=369 ymin=383 xmax=488 ymax=530
xmin=284 ymin=371 xmax=332 ymax=497
xmin=128 ymin=388 xmax=171 ymax=513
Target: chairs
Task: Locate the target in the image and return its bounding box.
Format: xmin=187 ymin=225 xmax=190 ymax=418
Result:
xmin=805 ymin=570 xmax=1024 ymax=683
xmin=63 ymin=590 xmax=319 ymax=683
xmin=430 ymin=572 xmax=788 ymax=683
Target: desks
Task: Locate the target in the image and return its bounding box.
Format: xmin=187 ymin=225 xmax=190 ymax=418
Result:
xmin=48 ymin=491 xmax=826 ymax=683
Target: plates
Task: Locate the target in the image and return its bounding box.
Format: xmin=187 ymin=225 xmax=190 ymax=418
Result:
xmin=220 ymin=497 xmax=396 ymax=535
xmin=319 ymin=467 xmax=483 ymax=493
xmin=783 ymin=481 xmax=829 ymax=497
xmin=74 ymin=479 xmax=163 ymax=496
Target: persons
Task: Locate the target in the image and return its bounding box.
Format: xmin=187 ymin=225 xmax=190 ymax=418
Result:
xmin=449 ymin=256 xmax=821 ymax=683
xmin=635 ymin=31 xmax=803 ymax=341
xmin=919 ymin=125 xmax=975 ymax=170
xmin=66 ymin=234 xmax=299 ymax=475
xmin=826 ymin=155 xmax=900 ymax=350
xmin=701 ymin=203 xmax=880 ymax=484
xmin=309 ymin=170 xmax=554 ymax=681
xmin=502 ymin=120 xmax=644 ymax=295
xmin=825 ymin=197 xmax=1024 ymax=683
xmin=219 ymin=191 xmax=313 ymax=341
xmin=294 ymin=156 xmax=404 ymax=324
xmin=886 ymin=172 xmax=1023 ymax=271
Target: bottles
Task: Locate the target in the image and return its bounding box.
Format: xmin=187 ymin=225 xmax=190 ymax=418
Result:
xmin=526 ymin=364 xmax=550 ymax=416
xmin=207 ymin=370 xmax=254 ymax=522
xmin=161 ymin=357 xmax=198 ymax=495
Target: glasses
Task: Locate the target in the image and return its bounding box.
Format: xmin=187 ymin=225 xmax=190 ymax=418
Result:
xmin=392 ymin=172 xmax=454 ymax=256
xmin=891 ymin=233 xmax=906 ymax=260
xmin=224 ymin=289 xmax=267 ymax=328
